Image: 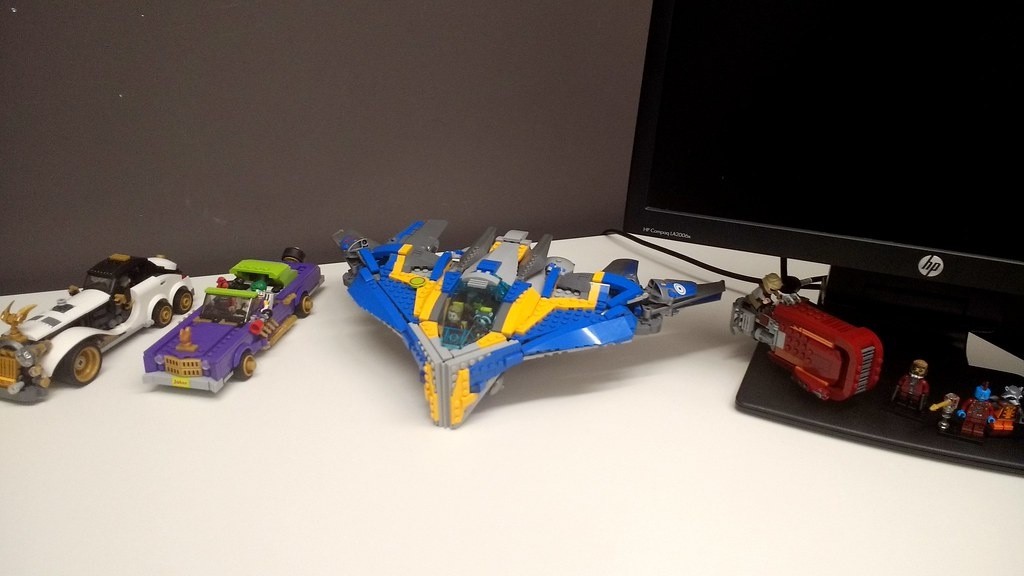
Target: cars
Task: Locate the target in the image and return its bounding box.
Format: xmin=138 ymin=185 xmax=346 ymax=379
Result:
xmin=143 ymin=246 xmax=325 ymax=394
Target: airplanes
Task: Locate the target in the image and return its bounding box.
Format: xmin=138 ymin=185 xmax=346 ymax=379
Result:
xmin=329 ymin=218 xmax=726 ymax=430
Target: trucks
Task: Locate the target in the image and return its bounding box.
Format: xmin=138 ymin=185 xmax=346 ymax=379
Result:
xmin=0 ymin=252 xmax=194 ymax=405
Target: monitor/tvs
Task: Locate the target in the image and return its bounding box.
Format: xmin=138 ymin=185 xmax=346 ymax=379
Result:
xmin=622 ymin=0 xmax=1024 ymax=482
